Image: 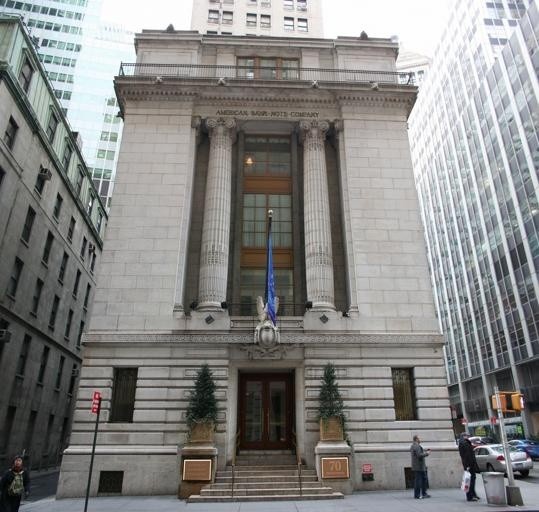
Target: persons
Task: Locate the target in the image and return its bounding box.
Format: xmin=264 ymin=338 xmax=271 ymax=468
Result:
xmin=0 ymin=455 xmax=30 ymax=512
xmin=411 ymin=435 xmax=431 ymax=499
xmin=458 ymin=432 xmax=480 ymax=501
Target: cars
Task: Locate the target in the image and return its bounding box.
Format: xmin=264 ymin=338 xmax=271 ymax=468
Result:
xmin=471 ymin=439 xmax=539 ymax=478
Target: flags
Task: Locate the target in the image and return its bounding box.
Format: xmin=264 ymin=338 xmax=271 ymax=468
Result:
xmin=265 ymin=224 xmax=276 ymax=325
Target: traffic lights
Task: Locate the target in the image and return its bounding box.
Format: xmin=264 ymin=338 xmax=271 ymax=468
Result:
xmin=519 ymin=396 xmax=524 ymax=409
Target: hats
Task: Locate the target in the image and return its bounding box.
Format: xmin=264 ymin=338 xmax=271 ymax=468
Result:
xmin=462 ymin=432 xmax=470 ymax=436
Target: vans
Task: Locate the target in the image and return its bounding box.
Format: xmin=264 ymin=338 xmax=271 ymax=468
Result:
xmin=468 ymin=436 xmax=493 ymax=445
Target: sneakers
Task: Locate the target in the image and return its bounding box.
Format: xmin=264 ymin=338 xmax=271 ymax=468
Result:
xmin=415 ymin=496 xmax=424 ymax=499
xmin=472 ymin=496 xmax=479 ymax=500
xmin=422 ymin=495 xmax=430 ymax=498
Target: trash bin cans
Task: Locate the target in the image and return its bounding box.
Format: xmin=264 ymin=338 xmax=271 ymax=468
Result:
xmin=483 ymin=472 xmax=505 ymax=504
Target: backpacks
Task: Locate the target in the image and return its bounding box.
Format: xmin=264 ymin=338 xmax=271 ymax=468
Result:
xmin=8 ymin=472 xmax=24 ymax=497
